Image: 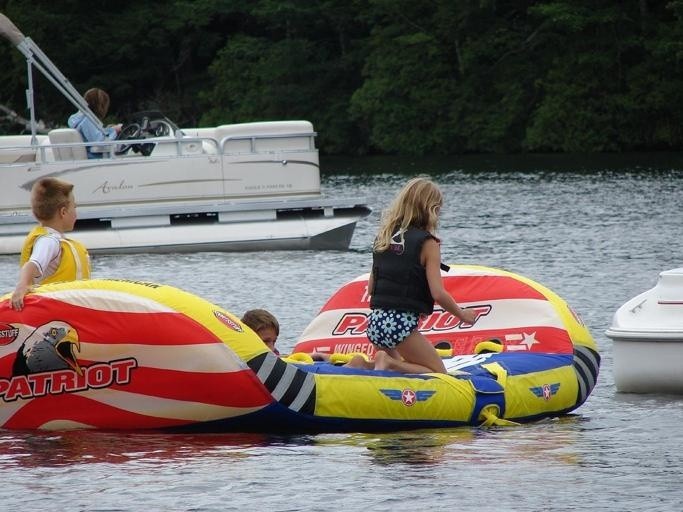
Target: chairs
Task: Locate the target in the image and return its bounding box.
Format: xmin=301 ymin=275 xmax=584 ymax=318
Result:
xmin=45 ymin=126 xmax=87 ymax=160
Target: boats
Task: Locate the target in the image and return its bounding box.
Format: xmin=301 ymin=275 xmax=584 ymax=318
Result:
xmin=0 ymin=14 xmax=378 ymax=257
xmin=603 ymin=268 xmax=682 ymax=393
xmin=0 ymin=263 xmax=601 ymax=435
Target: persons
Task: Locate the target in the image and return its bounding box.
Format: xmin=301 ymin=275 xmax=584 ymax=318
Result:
xmin=339 ymin=179 xmax=477 ymax=374
xmin=9 ymin=177 xmax=89 ymax=312
xmin=240 ymin=309 xmax=280 ymax=357
xmin=66 ymin=88 xmax=123 ymax=161
